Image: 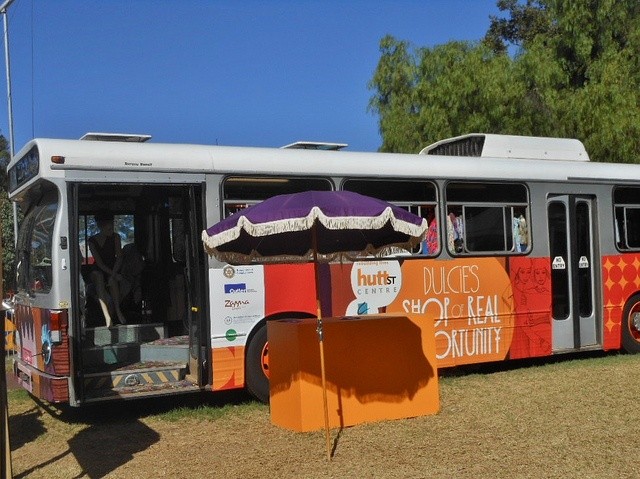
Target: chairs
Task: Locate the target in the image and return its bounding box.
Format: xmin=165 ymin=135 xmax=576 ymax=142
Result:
xmin=85 ymin=244 xmax=142 ymax=322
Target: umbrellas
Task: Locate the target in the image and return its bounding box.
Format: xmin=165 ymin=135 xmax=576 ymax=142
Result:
xmin=201 ymin=190 xmax=429 ymax=459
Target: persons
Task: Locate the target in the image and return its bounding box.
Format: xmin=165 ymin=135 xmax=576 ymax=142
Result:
xmin=88 ymin=212 xmax=127 ymax=328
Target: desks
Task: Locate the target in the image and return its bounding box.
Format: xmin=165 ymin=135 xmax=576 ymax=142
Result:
xmin=267 ymin=312 xmax=441 ymax=434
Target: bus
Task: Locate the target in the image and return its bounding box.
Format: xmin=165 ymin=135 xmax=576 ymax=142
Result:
xmin=6 ymin=133 xmax=640 ymax=406
